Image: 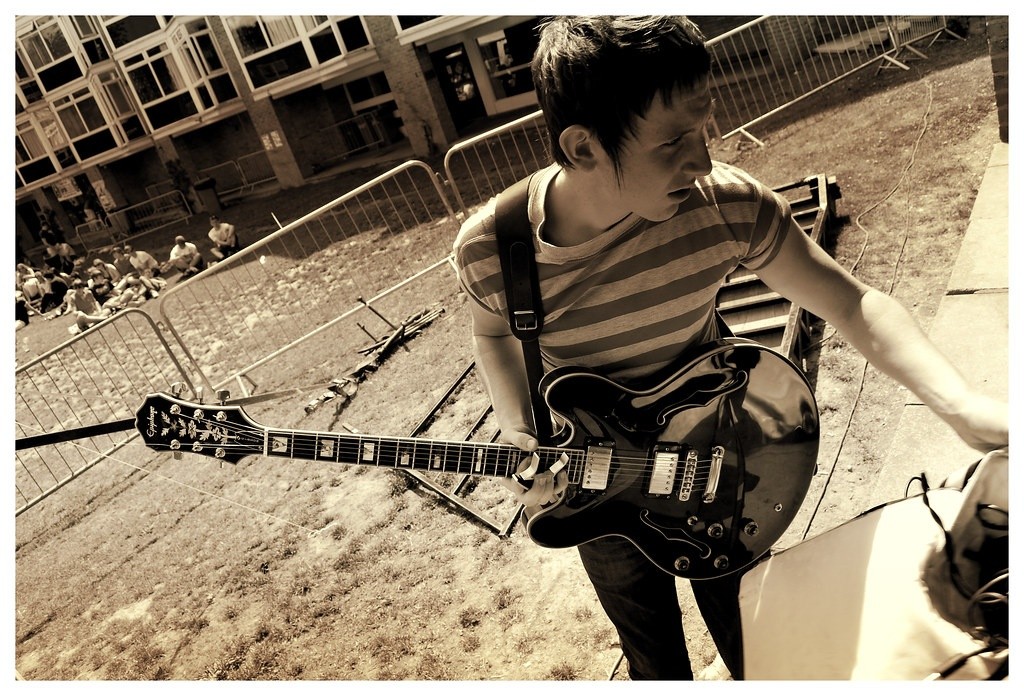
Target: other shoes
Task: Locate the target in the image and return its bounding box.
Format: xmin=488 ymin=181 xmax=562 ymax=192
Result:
xmin=149 ymin=289 xmax=160 ymax=300
xmin=133 ymin=296 xmax=146 ymax=308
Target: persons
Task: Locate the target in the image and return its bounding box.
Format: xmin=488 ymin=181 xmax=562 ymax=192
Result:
xmin=209 ymin=216 xmax=238 ymax=260
xmin=453 ymin=15 xmax=1008 ymax=681
xmin=169 ymin=236 xmax=204 ymax=278
xmin=16 ymin=220 xmax=165 ymax=331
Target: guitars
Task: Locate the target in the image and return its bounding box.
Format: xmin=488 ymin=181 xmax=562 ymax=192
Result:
xmin=133 ymin=336 xmax=824 ymax=583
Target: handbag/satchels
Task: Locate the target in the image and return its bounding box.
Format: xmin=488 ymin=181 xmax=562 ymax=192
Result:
xmin=922 ymin=443 xmax=1008 ymax=657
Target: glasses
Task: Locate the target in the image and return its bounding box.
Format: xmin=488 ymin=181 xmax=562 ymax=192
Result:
xmin=73 ymin=285 xmax=83 ymax=290
